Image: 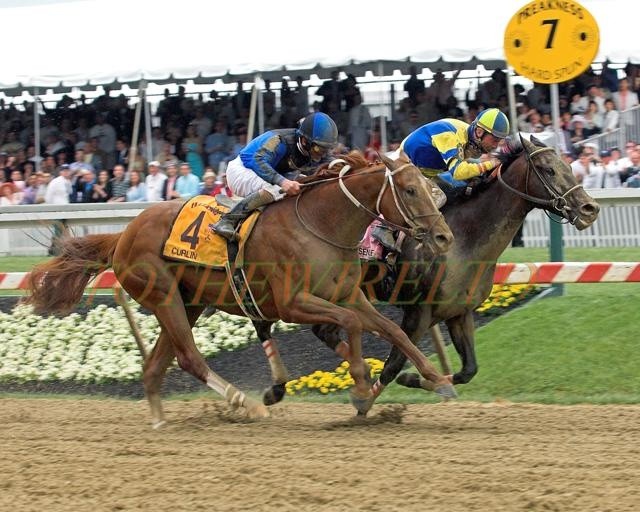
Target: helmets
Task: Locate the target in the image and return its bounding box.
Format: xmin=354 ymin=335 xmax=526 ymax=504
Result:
xmin=476 ymin=108 xmax=510 ymax=138
xmin=296 ymin=112 xmax=338 ymax=148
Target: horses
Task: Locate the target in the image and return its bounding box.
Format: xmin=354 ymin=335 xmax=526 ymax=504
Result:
xmin=26 ymin=148 xmax=455 ymax=431
xmin=251 ymin=133 xmax=601 ymax=419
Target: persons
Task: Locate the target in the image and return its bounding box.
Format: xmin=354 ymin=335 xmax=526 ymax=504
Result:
xmin=372 ymin=108 xmax=511 ymax=248
xmin=1 ymin=63 xmax=639 ymax=206
xmin=44 ymin=163 xmax=74 ymax=252
xmin=213 ymin=112 xmax=335 ymax=241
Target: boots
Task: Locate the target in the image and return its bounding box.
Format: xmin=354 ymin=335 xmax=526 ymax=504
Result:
xmin=213 ymin=202 xmax=251 ymax=241
xmin=372 ymin=223 xmax=395 ymax=248
